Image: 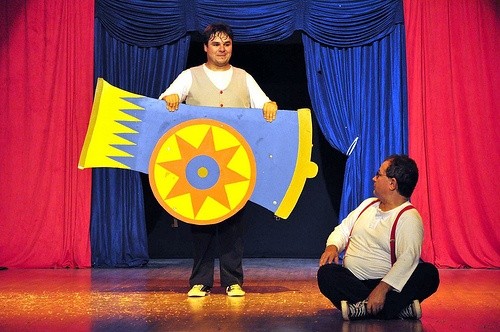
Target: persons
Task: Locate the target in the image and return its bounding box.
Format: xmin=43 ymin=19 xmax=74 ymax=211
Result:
xmin=317 ymin=154 xmax=439 ymax=321
xmin=157 ymin=25 xmax=279 ymax=296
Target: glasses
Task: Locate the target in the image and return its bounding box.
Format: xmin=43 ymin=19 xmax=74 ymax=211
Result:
xmin=376 ymin=171 xmax=392 ymax=180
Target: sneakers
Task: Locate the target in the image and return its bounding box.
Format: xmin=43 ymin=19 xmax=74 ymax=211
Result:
xmin=398 ymin=299 xmax=422 ymax=321
xmin=341 ymin=299 xmax=372 ymax=320
xmin=187 ymin=284 xmax=206 ymax=297
xmin=225 ymin=283 xmax=245 ymax=297
